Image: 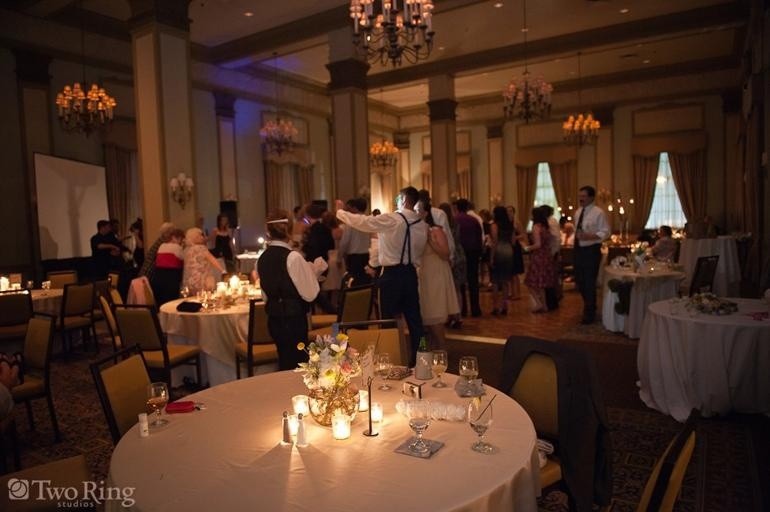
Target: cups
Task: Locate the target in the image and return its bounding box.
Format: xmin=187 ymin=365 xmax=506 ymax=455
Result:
xmin=371 ymin=402 xmax=382 ymax=422
xmin=331 ymin=413 xmax=352 ymax=439
xmin=358 ymin=389 xmax=368 ymax=412
xmin=42 ymin=281 xmax=50 ymax=296
xmin=415 ymin=351 xmax=434 ymax=380
xmin=291 ymin=394 xmax=311 ymax=417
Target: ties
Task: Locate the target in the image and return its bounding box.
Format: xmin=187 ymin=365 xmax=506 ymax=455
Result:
xmin=574 ymin=209 xmax=584 ymax=249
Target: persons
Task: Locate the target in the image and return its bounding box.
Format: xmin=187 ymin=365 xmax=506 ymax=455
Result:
xmin=253 ymin=209 xmax=321 ymax=371
xmin=561 ymin=223 xmax=575 ymax=283
xmin=335 ymin=185 xmax=429 ymax=370
xmin=89 ymin=198 xmax=561 ymax=355
xmin=417 ymin=189 xmax=457 ymax=271
xmin=648 ymin=226 xmax=677 ymax=262
xmin=573 ymin=185 xmax=612 ymax=323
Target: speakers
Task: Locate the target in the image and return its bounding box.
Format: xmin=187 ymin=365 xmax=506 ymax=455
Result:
xmin=220 ymin=200 xmax=238 ymax=229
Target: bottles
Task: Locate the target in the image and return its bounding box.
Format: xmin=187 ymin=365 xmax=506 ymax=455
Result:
xmin=281 ymin=410 xmax=294 ymax=445
xmin=416 ymin=335 xmax=428 ymax=351
xmin=295 ymin=412 xmax=310 ymax=448
xmin=138 ymin=413 xmax=150 ymax=437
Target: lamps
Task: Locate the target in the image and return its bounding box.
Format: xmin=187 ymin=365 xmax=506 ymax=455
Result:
xmin=499 ymin=1 xmax=554 ymax=124
xmin=171 ymin=172 xmax=195 ymax=207
xmin=358 ymin=183 xmax=370 ymax=199
xmin=260 ymin=48 xmax=298 ymax=155
xmin=347 ymin=0 xmax=435 ymax=68
xmin=558 ymin=51 xmax=600 ymax=149
xmin=56 ymin=0 xmax=117 ymax=138
xmin=490 ymin=192 xmax=504 ymax=205
xmin=372 ymin=89 xmax=399 ymax=171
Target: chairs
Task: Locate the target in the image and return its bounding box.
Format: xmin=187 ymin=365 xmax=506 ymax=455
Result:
xmin=1 ymin=219 xmax=770 ymax=512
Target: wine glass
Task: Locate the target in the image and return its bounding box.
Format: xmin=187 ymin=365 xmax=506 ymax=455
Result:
xmin=377 ymin=352 xmax=393 ymax=390
xmin=183 ymin=277 xmax=250 ymax=310
xmin=467 ymin=396 xmax=495 ymax=452
xmin=406 ymin=397 xmax=432 ymax=452
xmin=457 ymin=355 xmax=479 ymax=396
xmin=144 ymin=380 xmax=171 ymax=428
xmin=431 ymin=350 xmax=448 ymax=389
